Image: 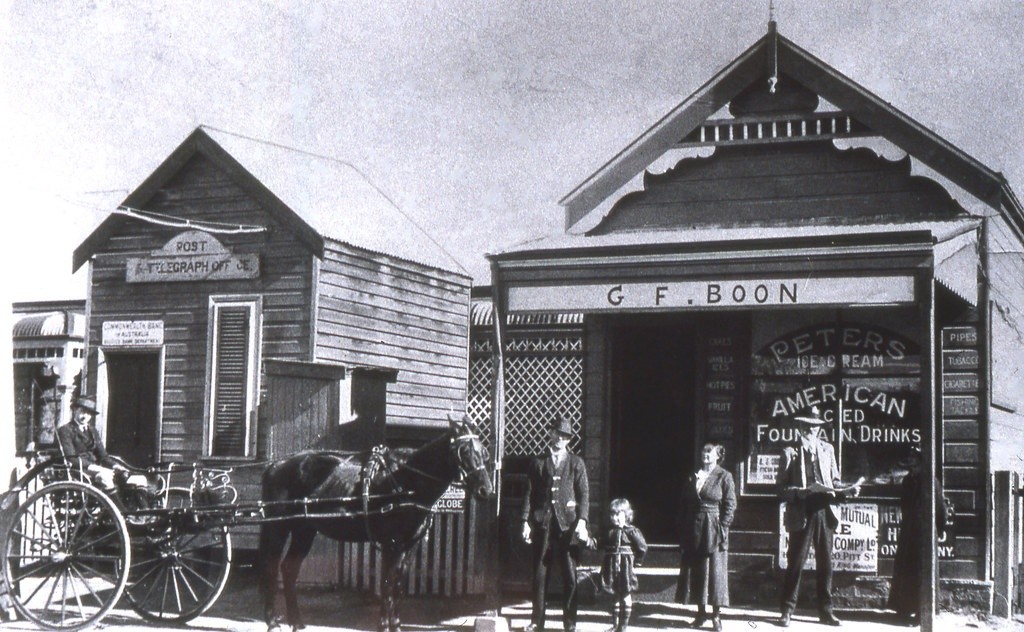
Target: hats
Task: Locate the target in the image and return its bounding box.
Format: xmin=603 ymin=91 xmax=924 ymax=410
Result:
xmin=69 ymin=397 xmax=100 ymax=415
xmin=548 ymin=419 xmax=574 ymax=437
xmin=793 ymin=404 xmax=826 ymax=424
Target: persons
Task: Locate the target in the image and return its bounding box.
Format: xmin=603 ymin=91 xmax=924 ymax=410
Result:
xmin=887 ymin=443 xmax=950 ymax=624
xmin=673 ymin=439 xmax=737 ymax=632
xmin=50 ymin=394 xmax=160 ymax=523
xmin=519 ymin=418 xmax=646 ymax=632
xmin=775 ymin=405 xmax=861 ymax=626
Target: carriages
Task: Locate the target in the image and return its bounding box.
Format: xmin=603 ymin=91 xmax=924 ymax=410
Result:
xmin=0 ymin=410 xmax=497 ymax=632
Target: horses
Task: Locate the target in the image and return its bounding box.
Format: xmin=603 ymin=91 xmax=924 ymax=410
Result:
xmin=257 ymin=414 xmax=493 ymax=632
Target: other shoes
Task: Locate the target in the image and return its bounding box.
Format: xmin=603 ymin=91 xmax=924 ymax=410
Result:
xmin=689 ymin=615 xmax=708 ymax=628
xmin=711 ymin=610 xmax=723 ymax=632
xmin=523 ymin=622 xmax=545 ymax=632
xmin=778 ymin=612 xmax=791 ymax=627
xmin=818 ymin=610 xmax=840 ymax=625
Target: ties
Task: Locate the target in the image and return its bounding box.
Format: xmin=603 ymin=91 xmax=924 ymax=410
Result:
xmin=807 ymin=445 xmax=819 ymax=483
xmin=83 ymin=426 xmax=86 ymax=432
xmin=555 ymin=454 xmax=560 ymax=468
